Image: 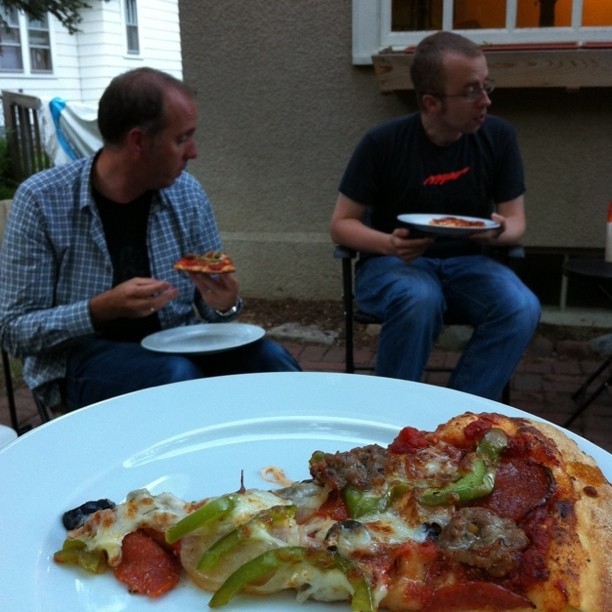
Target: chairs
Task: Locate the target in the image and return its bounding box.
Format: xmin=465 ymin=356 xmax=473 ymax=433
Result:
xmin=2 ymin=319 xmax=44 ymax=438
xmin=561 ymin=357 xmax=611 ymax=427
xmin=332 ymin=241 xmax=514 ymax=406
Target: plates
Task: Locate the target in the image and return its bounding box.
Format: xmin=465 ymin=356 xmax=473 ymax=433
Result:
xmin=141 ymin=323 xmax=265 ymax=356
xmin=0 ymin=369 xmax=612 ymax=611
xmin=398 ymin=212 xmax=502 ymax=236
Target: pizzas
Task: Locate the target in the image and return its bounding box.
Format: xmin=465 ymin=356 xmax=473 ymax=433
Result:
xmin=54 ymin=411 xmax=610 ymax=611
xmin=173 ymin=252 xmax=235 ymax=275
xmin=431 ymin=216 xmax=484 ymax=231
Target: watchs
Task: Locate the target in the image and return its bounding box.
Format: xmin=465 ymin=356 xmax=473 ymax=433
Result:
xmin=221 ymin=306 xmax=238 ymax=320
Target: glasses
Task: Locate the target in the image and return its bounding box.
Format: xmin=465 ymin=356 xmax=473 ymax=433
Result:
xmin=443 ymin=79 xmax=496 ymax=99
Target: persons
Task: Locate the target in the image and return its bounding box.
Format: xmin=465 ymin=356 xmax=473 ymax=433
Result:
xmin=329 ymin=32 xmax=543 ymax=399
xmin=0 ymin=66 xmax=302 ymax=427
